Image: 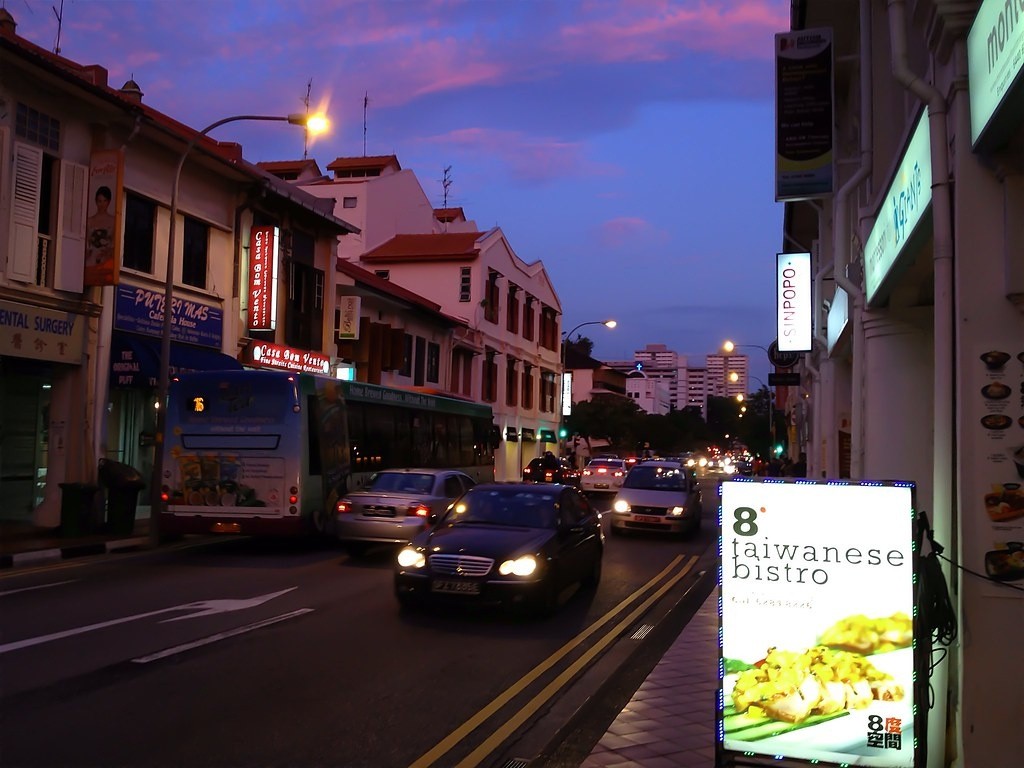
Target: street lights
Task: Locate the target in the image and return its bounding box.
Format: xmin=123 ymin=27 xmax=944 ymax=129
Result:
xmin=559 ymin=319 xmax=619 ymax=455
xmin=724 ymin=338 xmax=774 ymax=477
xmin=148 ymin=108 xmax=333 ymax=537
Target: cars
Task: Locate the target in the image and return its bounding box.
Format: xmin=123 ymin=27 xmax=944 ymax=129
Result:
xmin=521 ymin=451 xmax=754 ymax=493
xmin=393 ymin=481 xmax=605 ymax=618
xmin=608 ymin=460 xmax=705 ymax=539
xmin=333 ymin=466 xmax=484 ymax=555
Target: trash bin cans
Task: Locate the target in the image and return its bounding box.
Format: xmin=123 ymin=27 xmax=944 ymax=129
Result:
xmin=98 ymin=457 xmax=143 ymax=539
xmin=58 ymin=481 xmax=106 ymax=558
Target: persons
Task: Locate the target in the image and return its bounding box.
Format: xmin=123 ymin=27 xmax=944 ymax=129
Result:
xmin=88 ymin=186 xmax=116 ymax=258
xmin=750 ymin=451 xmax=807 ymax=480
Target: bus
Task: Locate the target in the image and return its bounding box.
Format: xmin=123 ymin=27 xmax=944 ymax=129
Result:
xmin=154 ymin=369 xmax=500 ymax=551
